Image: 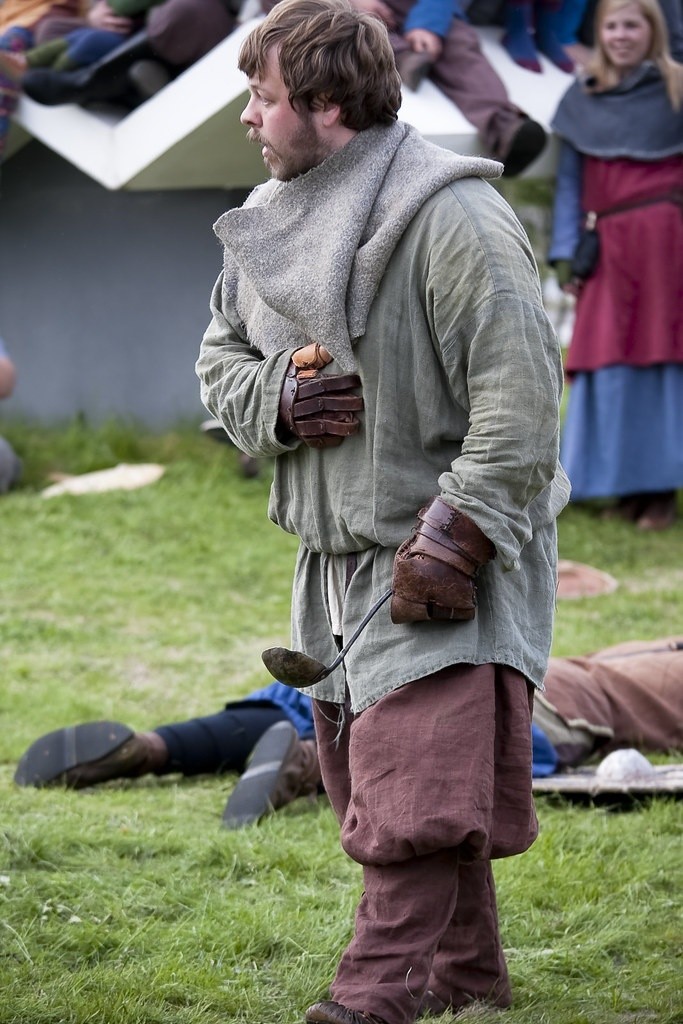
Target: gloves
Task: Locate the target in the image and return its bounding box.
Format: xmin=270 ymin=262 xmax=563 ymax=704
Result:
xmin=278 ymin=342 xmax=364 ymax=449
xmin=390 ymin=497 xmax=497 ymax=622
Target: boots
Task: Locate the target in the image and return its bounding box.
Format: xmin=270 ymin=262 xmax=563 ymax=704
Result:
xmin=20 ymin=32 xmax=174 ymax=107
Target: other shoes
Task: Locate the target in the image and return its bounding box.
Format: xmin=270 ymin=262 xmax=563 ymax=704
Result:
xmin=219 ymin=719 xmax=323 ymax=832
xmin=306 ymin=1001 xmax=389 ymax=1024
xmin=601 ymin=497 xmax=637 ymax=524
xmin=415 ymin=990 xmax=457 ymax=1019
xmin=638 ymin=497 xmax=674 ymax=529
xmin=492 ymin=106 xmax=546 ymax=178
xmin=389 ymin=32 xmax=428 ymax=92
xmin=14 ymin=720 xmax=157 ymax=788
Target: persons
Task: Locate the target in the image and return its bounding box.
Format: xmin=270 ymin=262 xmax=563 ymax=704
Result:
xmin=1 ymin=339 xmax=24 ymax=496
xmin=12 ymin=633 xmax=683 ymax=830
xmin=0 ymin=0 xmax=682 ymax=180
xmin=547 ymin=0 xmax=683 ymax=530
xmin=195 ymin=0 xmax=576 ymax=1024
